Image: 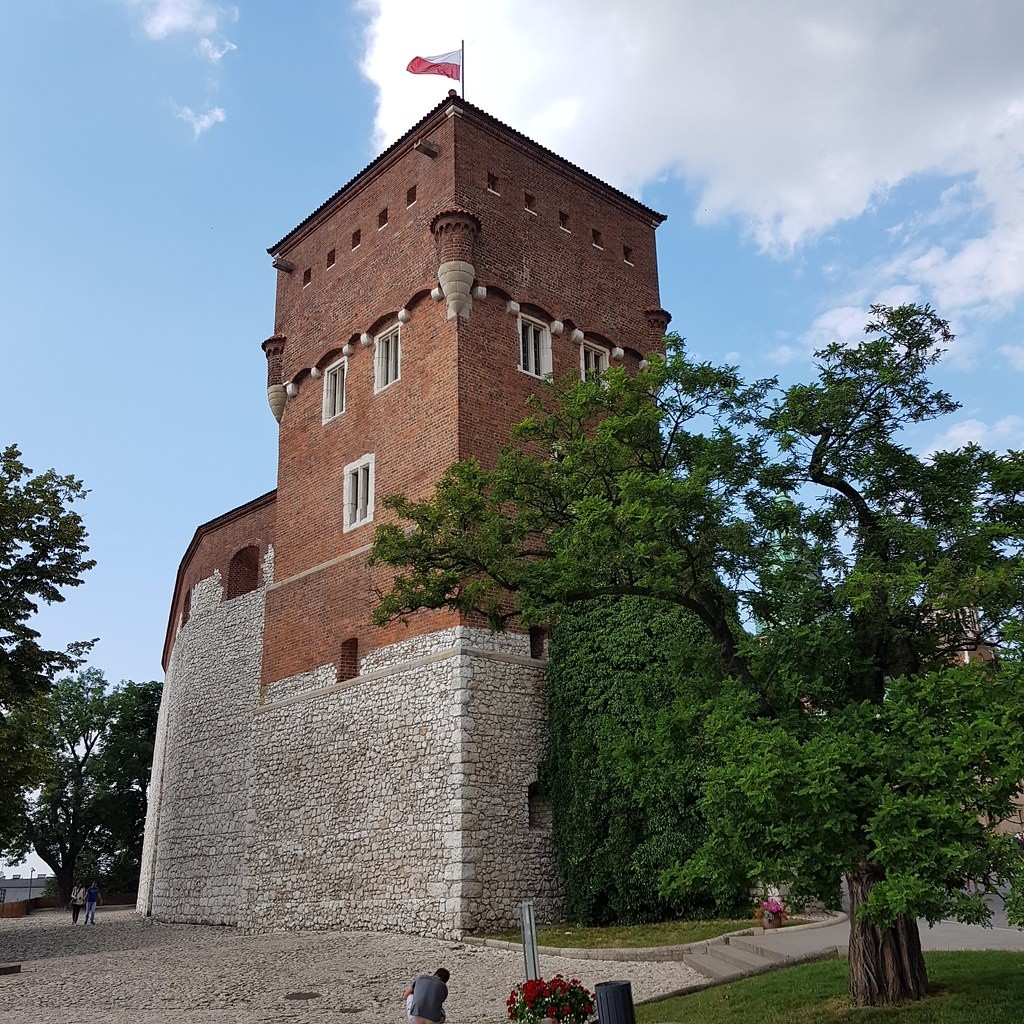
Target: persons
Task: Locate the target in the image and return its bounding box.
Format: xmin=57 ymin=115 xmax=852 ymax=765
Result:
xmin=84 ymin=881 xmax=103 ymax=924
xmin=403 ymin=968 xmax=449 ymax=1024
xmin=71 ymin=880 xmax=86 ymax=926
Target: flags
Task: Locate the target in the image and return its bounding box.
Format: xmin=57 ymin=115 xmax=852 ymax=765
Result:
xmin=407 ymin=48 xmax=460 ymax=80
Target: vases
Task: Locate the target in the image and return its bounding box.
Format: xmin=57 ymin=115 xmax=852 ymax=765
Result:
xmin=762 ymin=917 xmax=782 ymax=929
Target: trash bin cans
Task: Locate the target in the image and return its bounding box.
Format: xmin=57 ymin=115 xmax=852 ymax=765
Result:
xmin=594 ymin=979 xmax=638 ymax=1024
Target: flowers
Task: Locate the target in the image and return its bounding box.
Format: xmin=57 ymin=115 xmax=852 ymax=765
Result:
xmin=506 ymin=973 xmax=596 ymax=1024
xmin=752 ymin=898 xmax=790 ymax=924
